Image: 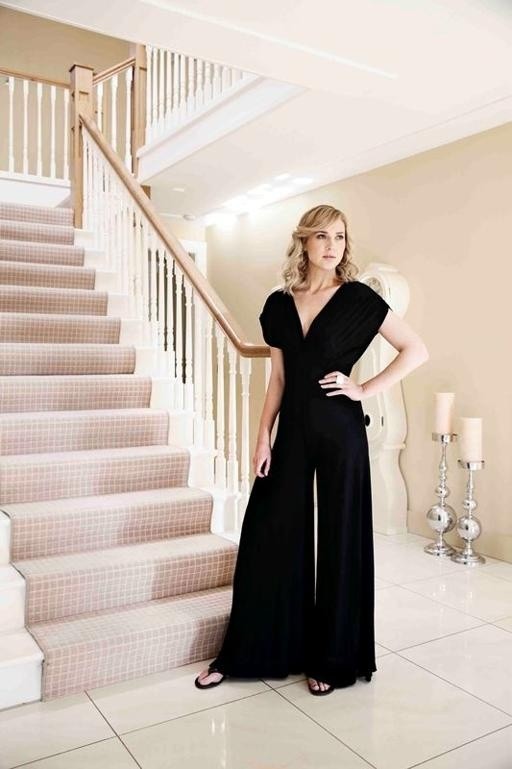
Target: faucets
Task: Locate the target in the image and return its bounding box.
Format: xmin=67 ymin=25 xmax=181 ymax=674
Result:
xmin=195 ymin=668 xmax=224 ymax=689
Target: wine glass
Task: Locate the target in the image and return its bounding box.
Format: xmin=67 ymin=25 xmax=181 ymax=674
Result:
xmin=334 ymin=374 xmax=344 ymax=386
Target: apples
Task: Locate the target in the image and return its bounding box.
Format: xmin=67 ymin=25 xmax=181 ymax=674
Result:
xmin=460 ymin=417 xmax=485 ymax=463
xmin=436 ymin=391 xmax=455 ymax=434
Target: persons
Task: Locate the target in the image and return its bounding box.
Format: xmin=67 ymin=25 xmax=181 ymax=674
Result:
xmin=195 ymin=202 xmax=431 ymax=699
